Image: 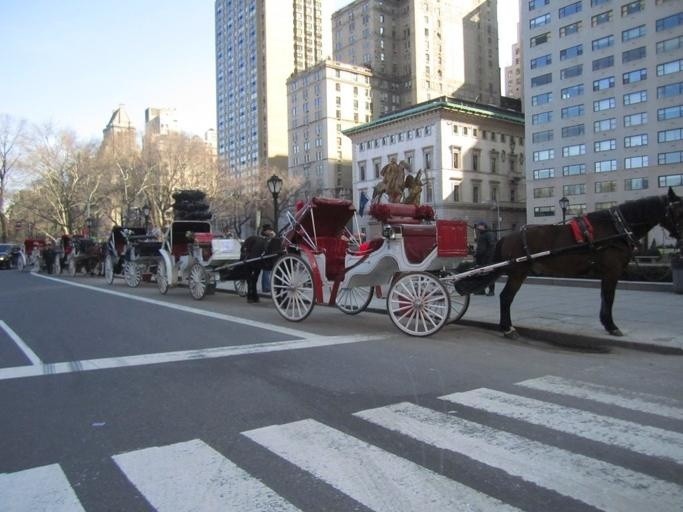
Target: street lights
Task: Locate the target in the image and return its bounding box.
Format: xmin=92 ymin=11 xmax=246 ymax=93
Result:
xmin=267 ymin=173 xmax=283 ymax=233
xmin=86 ymin=217 xmax=92 ymax=239
xmin=558 ymin=196 xmax=570 ymax=225
xmin=142 ymin=204 xmax=151 ymax=233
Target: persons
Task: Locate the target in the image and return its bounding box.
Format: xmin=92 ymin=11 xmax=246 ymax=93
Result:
xmin=32 ymin=244 xmax=56 ymax=274
xmin=473 ymin=222 xmax=497 ymax=296
xmin=260 ymin=224 xmax=276 ymax=293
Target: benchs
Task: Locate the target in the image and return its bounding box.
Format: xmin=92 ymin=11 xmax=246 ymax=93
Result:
xmin=307 ymin=236 xmax=348 ymax=280
xmin=172 ymin=234 xmax=241 ymax=268
xmin=396 ymin=224 xmax=436 ymax=261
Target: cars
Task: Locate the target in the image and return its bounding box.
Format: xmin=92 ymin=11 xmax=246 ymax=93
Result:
xmin=0 ymin=244 xmax=26 ymax=270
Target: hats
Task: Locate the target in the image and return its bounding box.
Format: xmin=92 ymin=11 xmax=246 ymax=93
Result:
xmin=261 ymin=224 xmax=273 ymax=236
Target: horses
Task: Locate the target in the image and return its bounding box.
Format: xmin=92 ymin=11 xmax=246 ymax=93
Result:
xmin=486 ymin=185 xmax=683 ymax=342
xmin=87 ymin=244 xmax=109 ymax=276
xmin=371 ymin=159 xmax=411 ymax=204
xmin=238 ymin=234 xmax=302 ymax=310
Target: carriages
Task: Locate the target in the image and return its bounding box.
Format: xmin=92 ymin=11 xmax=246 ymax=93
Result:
xmin=269 ymin=185 xmax=682 ymax=340
xmin=156 ymin=220 xmax=302 ymax=309
